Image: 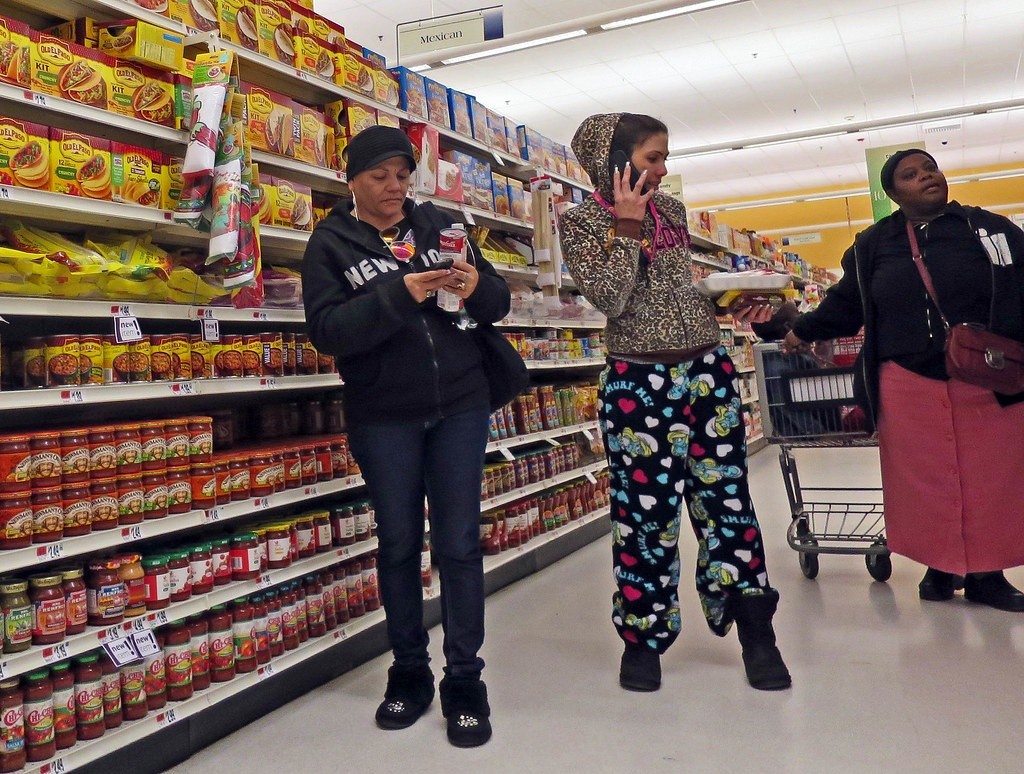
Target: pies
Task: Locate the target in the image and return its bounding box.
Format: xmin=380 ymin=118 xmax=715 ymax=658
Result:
xmin=0 ymin=1 xmax=398 ymax=233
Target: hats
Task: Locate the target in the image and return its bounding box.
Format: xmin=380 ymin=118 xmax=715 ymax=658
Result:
xmin=881 ymin=148 xmax=938 ymax=191
xmin=346 ymin=125 xmax=416 ymax=182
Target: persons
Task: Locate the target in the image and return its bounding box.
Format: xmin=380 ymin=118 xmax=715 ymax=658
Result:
xmin=301 ymin=124 xmax=512 ymax=748
xmin=751 ymin=149 xmax=1024 ymax=612
xmin=558 ymin=112 xmax=792 ymax=691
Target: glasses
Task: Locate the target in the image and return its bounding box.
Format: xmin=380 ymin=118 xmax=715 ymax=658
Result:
xmin=379 ymin=226 xmax=415 ymax=263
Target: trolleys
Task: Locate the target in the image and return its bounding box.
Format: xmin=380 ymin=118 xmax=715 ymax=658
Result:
xmin=752 ymin=326 xmax=894 ymax=582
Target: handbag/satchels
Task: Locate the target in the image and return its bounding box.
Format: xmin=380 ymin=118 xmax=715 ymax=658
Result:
xmin=471 ymin=315 xmax=531 ymax=412
xmin=944 ymin=324 xmax=1024 ymax=395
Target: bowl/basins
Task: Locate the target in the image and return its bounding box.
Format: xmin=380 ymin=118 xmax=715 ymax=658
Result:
xmin=695 ymin=271 xmax=791 ymax=297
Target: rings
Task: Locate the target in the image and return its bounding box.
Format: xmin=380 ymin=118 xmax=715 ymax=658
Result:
xmin=742 ymin=317 xmax=750 ymax=323
xmin=457 ymin=282 xmax=468 ymax=290
xmin=426 ymin=290 xmax=434 ymax=297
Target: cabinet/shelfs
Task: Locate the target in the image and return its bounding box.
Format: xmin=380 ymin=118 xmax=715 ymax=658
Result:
xmin=0 ymin=0 xmax=838 ymax=774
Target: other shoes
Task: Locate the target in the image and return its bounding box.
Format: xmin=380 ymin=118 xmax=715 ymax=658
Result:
xmin=439 ymin=676 xmax=492 ymax=748
xmin=744 ymin=646 xmax=792 ymax=690
xmin=619 ymin=645 xmax=662 ymax=692
xmin=965 ymin=570 xmax=1024 ymax=613
xmin=920 ymin=566 xmax=965 ymax=602
xmin=375 ymin=665 xmax=435 ymax=731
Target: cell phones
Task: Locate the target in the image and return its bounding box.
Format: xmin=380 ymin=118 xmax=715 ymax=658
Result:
xmin=428 ymin=257 xmax=454 ymax=270
xmin=608 ymin=149 xmax=649 ymax=196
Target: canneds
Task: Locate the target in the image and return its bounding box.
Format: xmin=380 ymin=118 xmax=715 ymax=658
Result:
xmin=0 ymin=329 xmax=608 ymax=774
